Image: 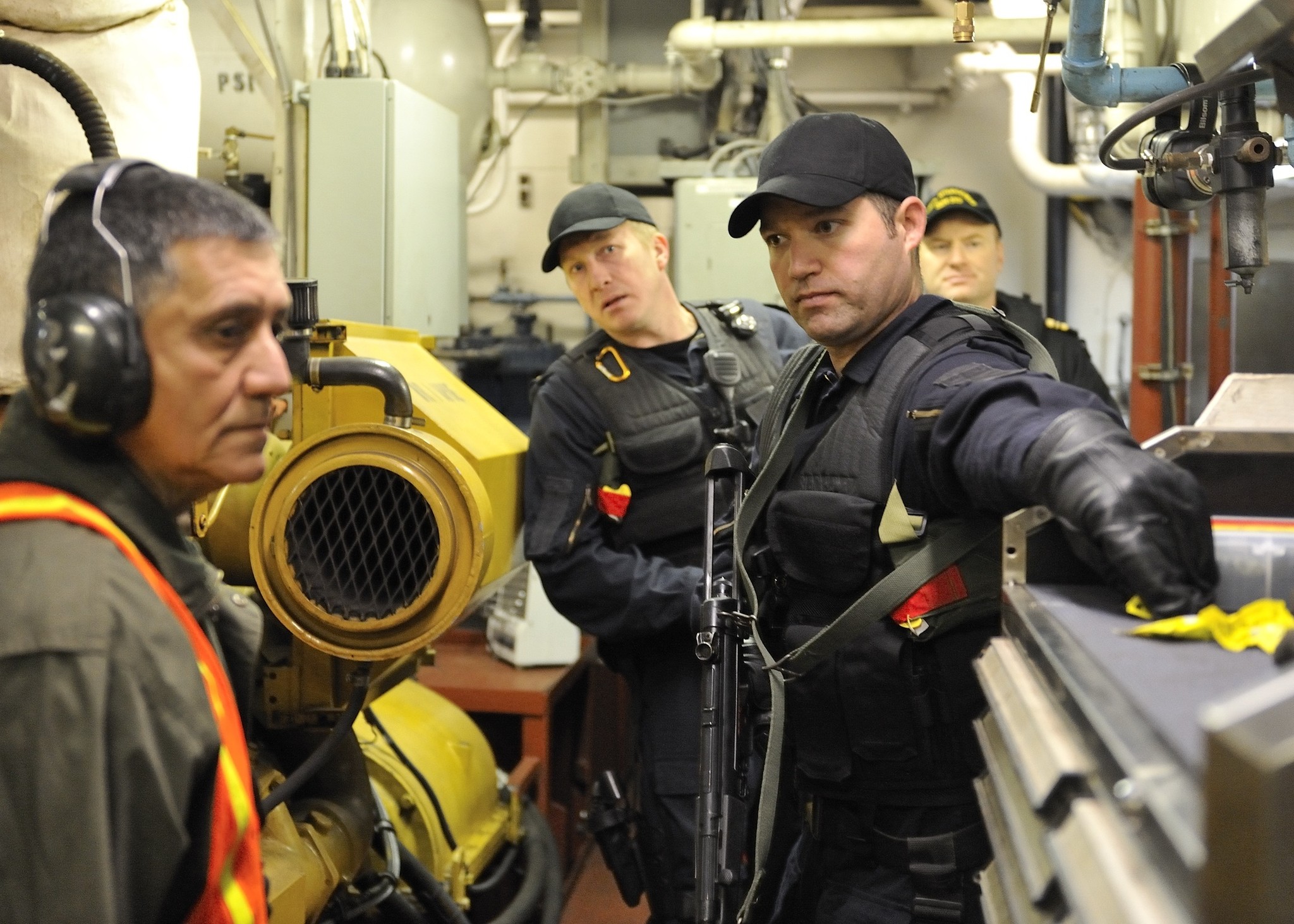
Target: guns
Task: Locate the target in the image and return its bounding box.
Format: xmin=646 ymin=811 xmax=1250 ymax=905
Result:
xmin=686 ymin=442 xmax=780 ymax=923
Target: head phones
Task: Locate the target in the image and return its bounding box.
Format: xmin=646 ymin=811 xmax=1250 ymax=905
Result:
xmin=21 ymin=158 xmax=164 ymax=440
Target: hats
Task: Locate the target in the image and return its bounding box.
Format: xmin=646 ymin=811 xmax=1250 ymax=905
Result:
xmin=728 ymin=112 xmax=915 ymax=238
xmin=925 ymin=186 xmax=1002 ymax=235
xmin=541 ymin=183 xmax=658 ymax=272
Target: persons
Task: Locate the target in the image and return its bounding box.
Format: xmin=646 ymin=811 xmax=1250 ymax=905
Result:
xmin=916 ymin=186 xmax=1123 ymax=427
xmin=1 ymin=159 xmax=295 ymax=924
xmin=522 ymin=114 xmax=1222 ymax=924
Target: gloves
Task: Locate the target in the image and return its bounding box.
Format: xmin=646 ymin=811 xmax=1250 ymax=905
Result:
xmin=1021 ymin=407 xmax=1220 ymax=616
